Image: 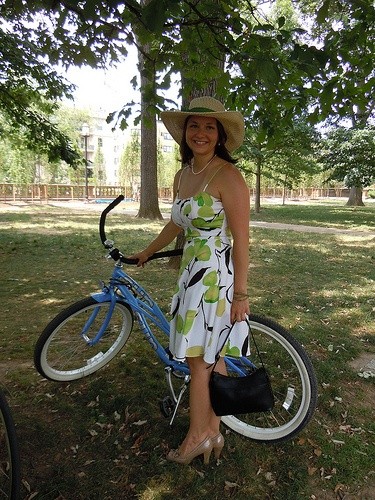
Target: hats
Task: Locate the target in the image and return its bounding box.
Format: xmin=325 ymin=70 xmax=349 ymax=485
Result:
xmin=159 ymin=96 xmax=246 ymax=153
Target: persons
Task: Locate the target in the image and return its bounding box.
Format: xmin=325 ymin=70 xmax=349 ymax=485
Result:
xmin=125 ymin=96 xmax=250 ymax=462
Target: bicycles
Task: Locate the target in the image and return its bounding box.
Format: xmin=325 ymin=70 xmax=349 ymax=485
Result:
xmin=31 ymin=194 xmax=317 ymax=445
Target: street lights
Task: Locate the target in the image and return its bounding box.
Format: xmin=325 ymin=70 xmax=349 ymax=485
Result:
xmin=81 ymin=123 xmax=90 ymax=200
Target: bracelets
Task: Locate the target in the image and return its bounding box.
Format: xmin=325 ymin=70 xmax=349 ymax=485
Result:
xmin=234 ymin=292 xmax=249 ymax=302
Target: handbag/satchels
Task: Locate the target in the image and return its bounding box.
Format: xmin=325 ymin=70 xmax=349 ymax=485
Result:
xmin=208 ymin=363 xmax=275 ymax=417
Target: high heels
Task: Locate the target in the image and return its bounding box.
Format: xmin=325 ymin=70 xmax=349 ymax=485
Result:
xmin=210 ymin=432 xmax=225 ymax=460
xmin=165 ymin=435 xmax=214 ymax=466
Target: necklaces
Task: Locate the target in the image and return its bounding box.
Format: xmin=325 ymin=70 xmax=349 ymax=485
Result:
xmin=191 ymin=154 xmax=216 ymax=174
